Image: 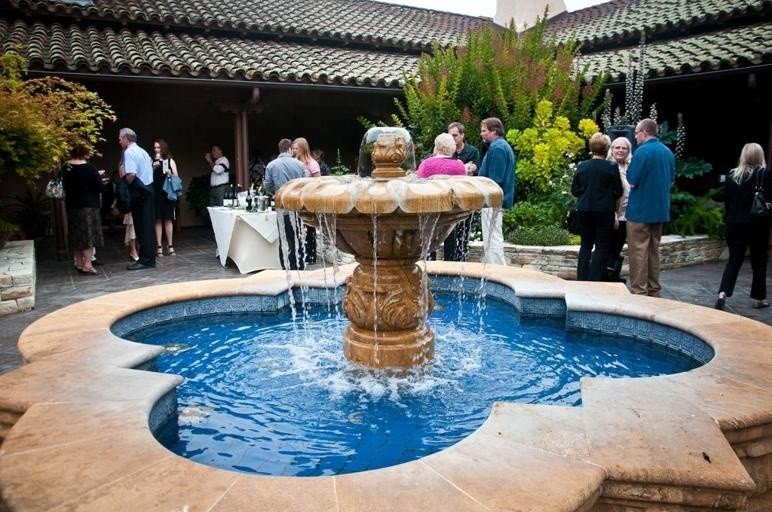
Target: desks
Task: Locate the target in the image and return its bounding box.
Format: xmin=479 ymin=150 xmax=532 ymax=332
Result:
xmin=207 ymin=205 xmax=305 ymax=275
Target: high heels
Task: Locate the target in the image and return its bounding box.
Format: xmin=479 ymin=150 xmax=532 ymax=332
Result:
xmin=129 ymin=251 xmax=140 ymax=261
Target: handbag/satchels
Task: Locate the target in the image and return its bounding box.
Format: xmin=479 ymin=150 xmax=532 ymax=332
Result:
xmin=749 ymin=166 xmax=770 ymax=217
xmin=566 ymin=206 xmax=581 ymax=234
xmin=45 ymin=177 xmax=66 ymax=200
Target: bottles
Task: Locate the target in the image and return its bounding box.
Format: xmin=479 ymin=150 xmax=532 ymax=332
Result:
xmin=224 ymin=183 xmax=274 ymax=210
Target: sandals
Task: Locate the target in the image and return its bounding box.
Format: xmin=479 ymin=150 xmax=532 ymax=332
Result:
xmin=156 ymin=244 xmax=165 ymax=257
xmin=164 ymin=244 xmax=177 ymax=256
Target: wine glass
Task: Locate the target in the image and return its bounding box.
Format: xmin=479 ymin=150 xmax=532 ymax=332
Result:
xmin=156 ymin=153 xmax=164 ymax=165
xmin=97 ymin=166 xmax=108 ymax=185
xmin=201 ymin=152 xmax=215 ymax=160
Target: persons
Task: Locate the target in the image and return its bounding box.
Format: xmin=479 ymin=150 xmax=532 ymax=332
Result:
xmin=204 ymin=141 xmax=232 ymax=244
xmin=476 ymin=118 xmax=517 ymax=268
xmin=417 ymin=132 xmax=467 ymax=261
xmin=625 ymin=116 xmax=679 ymax=297
xmin=601 ymin=135 xmax=634 ymax=280
xmin=569 ymin=131 xmax=623 ymax=281
xmin=62 ymin=127 xmax=182 ymax=276
xmin=293 ymin=135 xmax=322 ymax=266
xmin=709 ymin=143 xmax=772 ymax=313
xmin=264 ymin=138 xmax=306 ymax=271
xmin=442 ymin=121 xmax=481 ymax=260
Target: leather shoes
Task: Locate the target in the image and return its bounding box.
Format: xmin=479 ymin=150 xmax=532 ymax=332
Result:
xmin=717 ymin=291 xmax=727 ymax=308
xmin=752 ymin=299 xmax=771 ymax=309
xmin=127 ymin=262 xmax=155 ymax=270
xmin=73 ymin=258 xmax=103 ymax=276
